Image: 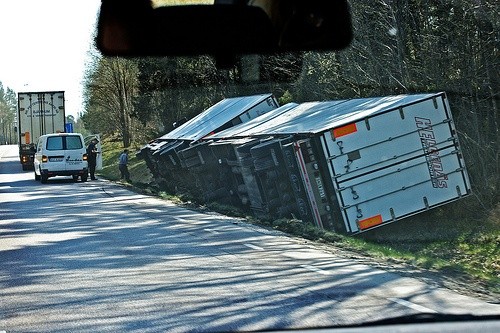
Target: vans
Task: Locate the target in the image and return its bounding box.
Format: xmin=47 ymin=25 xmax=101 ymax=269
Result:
xmin=36 ymin=132 xmax=90 ymax=184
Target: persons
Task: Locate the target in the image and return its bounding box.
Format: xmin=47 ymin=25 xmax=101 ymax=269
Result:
xmin=87 ymin=138 xmax=100 ymax=180
xmin=119 ymin=149 xmax=130 ymax=177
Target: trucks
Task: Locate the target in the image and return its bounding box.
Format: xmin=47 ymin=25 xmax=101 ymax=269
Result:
xmin=135 ymin=93 xmax=281 ymax=201
xmin=180 ymin=91 xmax=473 ymax=237
xmin=18 ymin=91 xmax=65 ymax=171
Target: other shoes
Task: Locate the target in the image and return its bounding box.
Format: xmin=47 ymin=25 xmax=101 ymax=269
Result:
xmin=91 ymin=177 xmax=97 ymax=180
